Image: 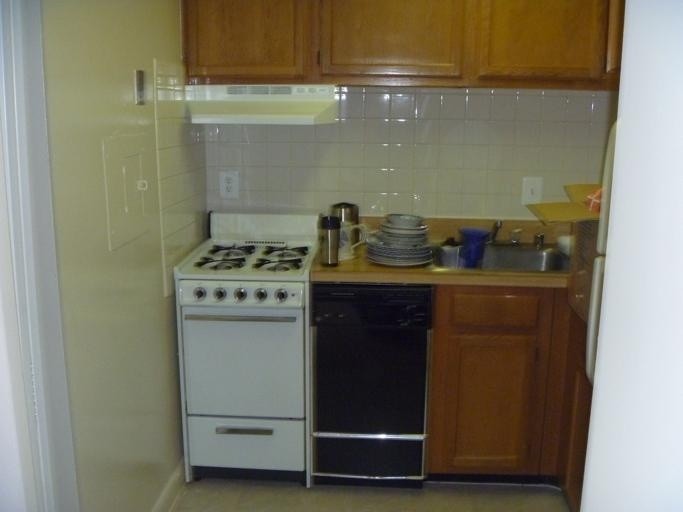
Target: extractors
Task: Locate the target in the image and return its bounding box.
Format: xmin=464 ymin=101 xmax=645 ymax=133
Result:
xmin=187 ymin=87 xmax=338 ymax=123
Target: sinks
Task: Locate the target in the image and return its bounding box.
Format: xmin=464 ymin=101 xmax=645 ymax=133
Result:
xmin=430 ymin=242 xmax=571 ymax=276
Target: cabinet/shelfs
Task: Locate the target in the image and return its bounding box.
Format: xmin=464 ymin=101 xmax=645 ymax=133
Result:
xmin=425 ymin=285 xmax=592 ymax=511
xmin=314 ymin=0 xmax=609 ymax=90
xmin=186 ymin=0 xmax=313 ymax=85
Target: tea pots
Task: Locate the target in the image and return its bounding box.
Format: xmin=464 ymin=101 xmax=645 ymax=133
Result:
xmin=460 ymin=227 xmax=491 ymax=268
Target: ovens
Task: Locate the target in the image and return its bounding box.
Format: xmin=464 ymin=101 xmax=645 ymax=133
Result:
xmin=179 ymin=307 xmax=309 ymax=421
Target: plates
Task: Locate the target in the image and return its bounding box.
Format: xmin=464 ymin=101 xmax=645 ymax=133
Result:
xmin=363 ymin=224 xmax=435 ymax=268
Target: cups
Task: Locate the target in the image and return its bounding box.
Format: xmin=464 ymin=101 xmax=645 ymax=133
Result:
xmin=314 ymin=215 xmax=341 ymax=266
xmin=337 ymin=219 xmax=364 ymax=260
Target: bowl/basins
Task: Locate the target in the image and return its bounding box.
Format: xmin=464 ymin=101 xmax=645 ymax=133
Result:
xmin=384 ymin=213 xmax=423 ymax=226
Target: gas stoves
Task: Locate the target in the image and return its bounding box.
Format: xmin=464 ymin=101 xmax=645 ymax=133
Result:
xmin=193 ymin=239 xmax=305 ymax=279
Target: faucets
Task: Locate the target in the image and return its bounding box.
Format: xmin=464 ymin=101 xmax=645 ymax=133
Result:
xmin=490 ymin=220 xmax=502 ymax=243
